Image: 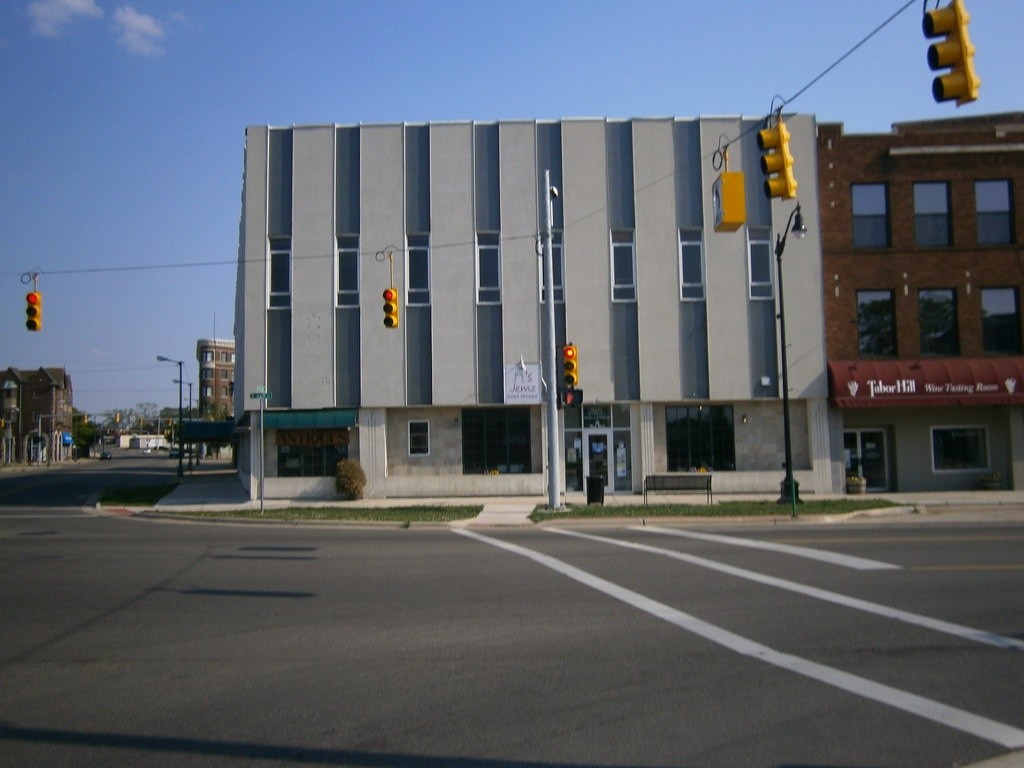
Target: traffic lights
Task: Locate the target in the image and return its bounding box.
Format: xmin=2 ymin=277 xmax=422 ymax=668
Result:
xmin=558 ymin=389 xmax=584 ymax=409
xmin=25 ymin=292 xmax=42 ymax=331
xmin=563 ymin=345 xmax=579 ymax=386
xmin=757 ymin=118 xmax=796 ymax=201
xmin=383 ymin=287 xmax=400 ymax=328
xmin=922 ymin=0 xmax=980 ymax=108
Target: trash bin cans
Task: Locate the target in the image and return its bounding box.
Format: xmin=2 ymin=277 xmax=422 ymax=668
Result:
xmin=585 ymin=476 xmax=604 ymax=507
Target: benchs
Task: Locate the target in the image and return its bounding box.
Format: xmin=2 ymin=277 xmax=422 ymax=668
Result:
xmin=642 ymin=475 xmax=714 ymax=506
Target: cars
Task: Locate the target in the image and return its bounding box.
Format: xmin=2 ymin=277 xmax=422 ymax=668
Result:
xmin=100 ymin=452 xmax=112 ymax=460
xmin=142 ymin=447 xmax=151 ymax=455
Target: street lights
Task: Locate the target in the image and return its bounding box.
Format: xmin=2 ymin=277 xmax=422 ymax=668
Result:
xmin=775 ymin=202 xmax=810 ymax=504
xmin=157 ymin=356 xmax=184 ymax=478
xmin=172 ymin=379 xmax=193 ymax=470
